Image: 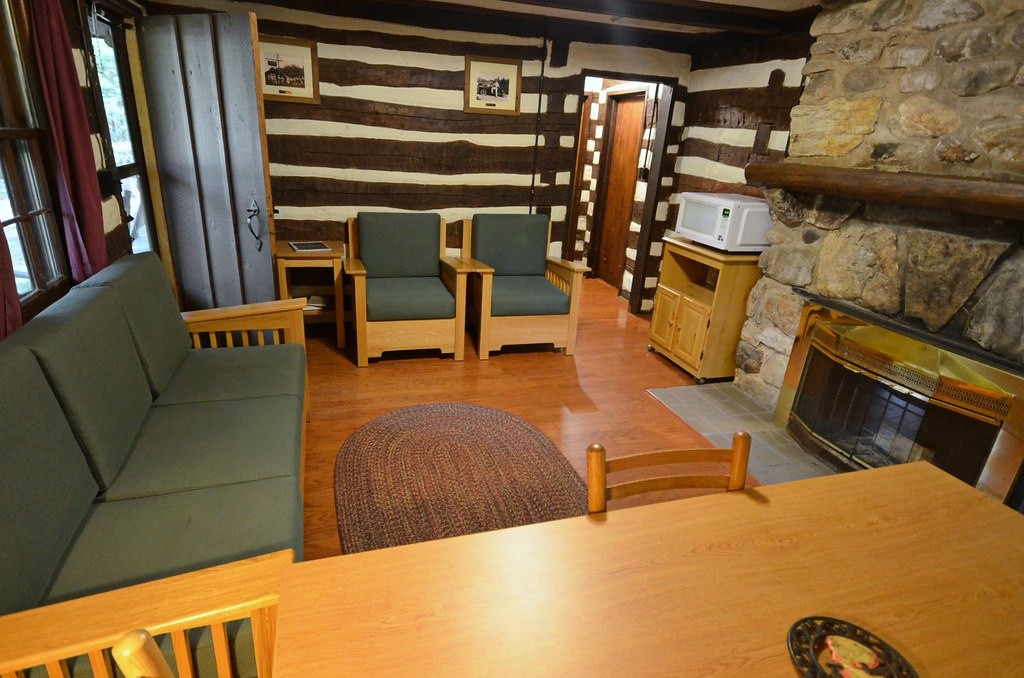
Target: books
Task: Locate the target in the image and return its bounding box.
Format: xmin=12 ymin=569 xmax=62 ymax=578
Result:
xmin=303 ymin=296 xmax=326 ymax=310
xmin=289 ymin=241 xmax=330 ymax=252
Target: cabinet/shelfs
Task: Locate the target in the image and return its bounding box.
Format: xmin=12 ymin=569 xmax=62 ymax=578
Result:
xmin=647 ymin=235 xmax=763 ymax=384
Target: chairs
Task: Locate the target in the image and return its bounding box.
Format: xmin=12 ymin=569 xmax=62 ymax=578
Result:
xmin=343 ymin=210 xmax=476 ymax=367
xmin=455 ymin=212 xmax=592 ymax=360
xmin=588 ymin=432 xmax=752 ymax=514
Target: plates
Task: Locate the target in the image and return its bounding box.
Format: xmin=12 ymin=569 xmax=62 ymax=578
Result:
xmin=786 ymin=615 xmax=921 ymax=678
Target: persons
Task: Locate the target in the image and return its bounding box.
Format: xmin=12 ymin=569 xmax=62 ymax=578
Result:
xmin=491 ymin=79 xmax=499 ymax=97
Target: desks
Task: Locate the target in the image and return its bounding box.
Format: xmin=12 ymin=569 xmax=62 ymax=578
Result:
xmin=274 ymin=239 xmax=345 ymax=348
xmin=272 ymin=460 xmax=1024 ymax=678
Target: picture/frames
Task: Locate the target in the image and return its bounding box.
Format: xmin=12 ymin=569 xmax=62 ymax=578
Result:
xmin=464 ymin=55 xmax=523 ymax=116
xmin=258 ymin=34 xmax=321 ymax=105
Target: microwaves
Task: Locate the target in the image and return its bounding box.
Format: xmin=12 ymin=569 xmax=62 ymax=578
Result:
xmin=675 ymin=192 xmax=772 ymax=252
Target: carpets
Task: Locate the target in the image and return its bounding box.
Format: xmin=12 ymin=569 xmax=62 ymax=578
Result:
xmin=334 ymin=403 xmax=588 ymax=555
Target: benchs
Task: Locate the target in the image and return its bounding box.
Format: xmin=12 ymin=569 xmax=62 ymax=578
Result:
xmin=0 ymin=250 xmax=309 ymax=678
